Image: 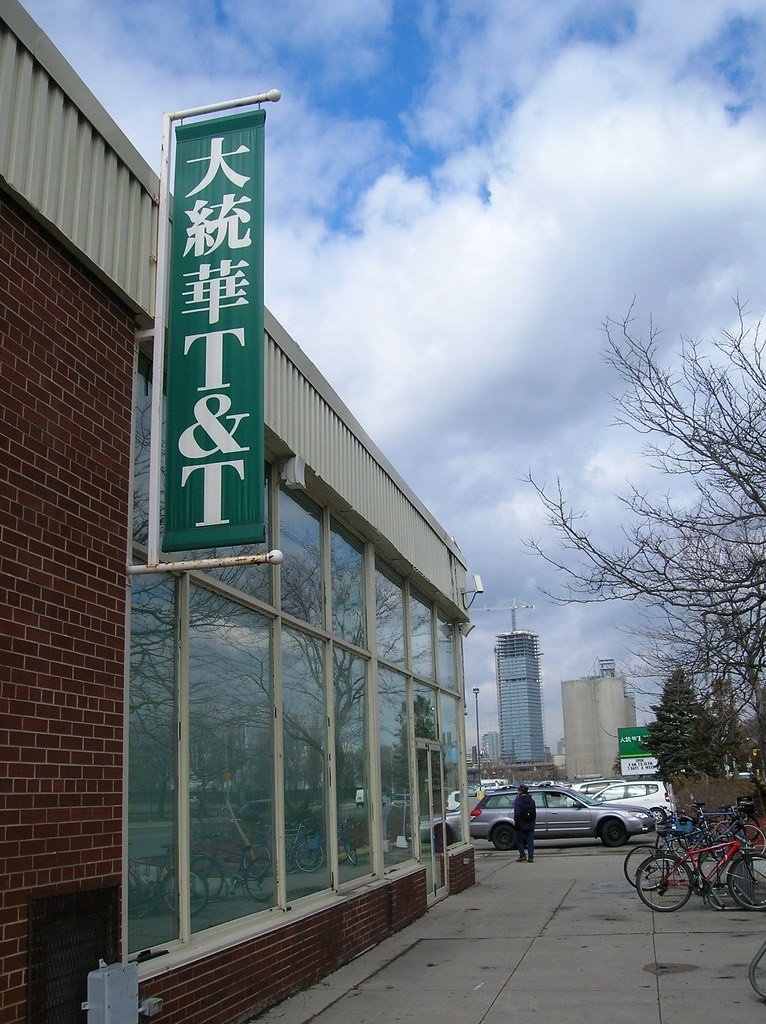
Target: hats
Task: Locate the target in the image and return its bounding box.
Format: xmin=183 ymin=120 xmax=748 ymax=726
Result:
xmin=519 ymin=785 xmax=528 ymax=792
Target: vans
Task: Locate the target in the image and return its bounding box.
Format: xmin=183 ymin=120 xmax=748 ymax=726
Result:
xmin=356 ymin=789 xmax=365 ymax=809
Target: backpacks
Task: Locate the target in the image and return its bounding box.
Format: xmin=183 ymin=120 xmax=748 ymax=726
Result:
xmin=518 ymin=797 xmax=535 ymax=824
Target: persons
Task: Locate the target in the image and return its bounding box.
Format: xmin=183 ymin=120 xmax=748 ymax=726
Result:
xmin=512 ymin=784 xmax=536 ymax=863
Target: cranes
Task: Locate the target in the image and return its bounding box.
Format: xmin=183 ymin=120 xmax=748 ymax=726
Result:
xmin=469 ymin=599 xmax=534 ymax=630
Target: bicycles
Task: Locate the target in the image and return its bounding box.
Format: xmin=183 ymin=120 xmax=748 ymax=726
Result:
xmin=624 ymin=793 xmax=766 ymax=912
xmin=128 ymin=815 xmax=360 ymax=919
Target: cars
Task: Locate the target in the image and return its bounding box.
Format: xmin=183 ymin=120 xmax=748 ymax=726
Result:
xmin=419 ymin=806 xmax=462 ymax=853
xmin=391 ymin=792 xmax=411 ymax=809
xmin=468 ymin=778 xmax=627 ymax=807
xmin=229 ymin=798 xmax=293 ymax=833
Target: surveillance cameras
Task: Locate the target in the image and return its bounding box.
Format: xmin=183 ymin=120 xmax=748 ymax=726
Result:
xmin=473 ymin=574 xmax=484 ymax=594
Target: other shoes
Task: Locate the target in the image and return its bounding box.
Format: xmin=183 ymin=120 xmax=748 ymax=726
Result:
xmin=516 ymin=856 xmax=527 ymax=862
xmin=528 ymin=859 xmax=533 ymax=862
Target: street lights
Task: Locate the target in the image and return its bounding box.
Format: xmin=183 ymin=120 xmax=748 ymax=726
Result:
xmin=473 ymin=686 xmax=482 ymax=791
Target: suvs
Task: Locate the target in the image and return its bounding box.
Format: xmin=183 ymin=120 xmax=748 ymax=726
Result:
xmin=592 ymin=781 xmax=672 ymax=825
xmin=468 ymin=784 xmax=657 ymax=851
